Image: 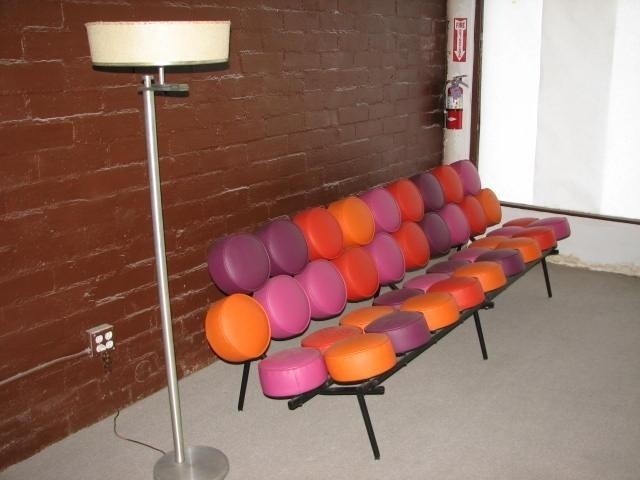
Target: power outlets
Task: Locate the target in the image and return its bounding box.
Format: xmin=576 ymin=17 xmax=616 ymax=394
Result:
xmin=85 ymin=323 xmax=117 ymax=360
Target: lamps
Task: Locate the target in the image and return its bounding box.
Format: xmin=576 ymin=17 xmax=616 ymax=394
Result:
xmin=83 ymin=19 xmax=233 ymax=480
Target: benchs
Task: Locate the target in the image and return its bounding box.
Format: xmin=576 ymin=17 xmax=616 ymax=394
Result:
xmin=203 ymin=160 xmax=571 ymax=459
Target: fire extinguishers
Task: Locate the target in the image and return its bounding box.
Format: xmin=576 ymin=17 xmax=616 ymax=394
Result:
xmin=443 ymin=75 xmax=469 ymax=129
